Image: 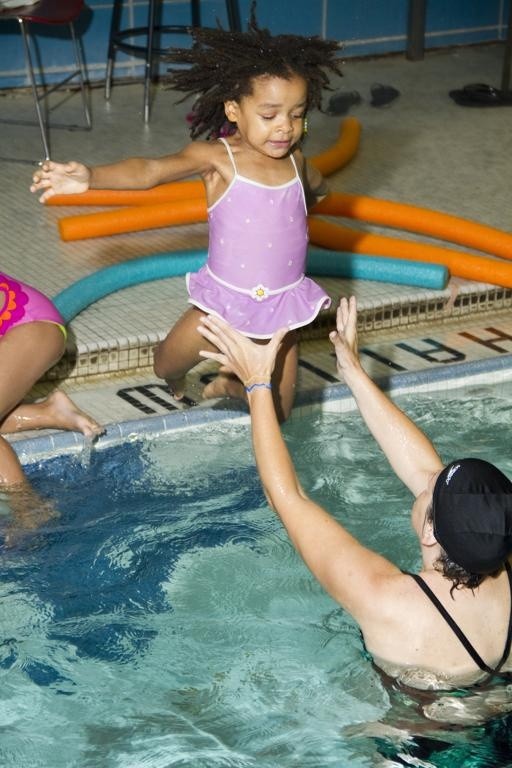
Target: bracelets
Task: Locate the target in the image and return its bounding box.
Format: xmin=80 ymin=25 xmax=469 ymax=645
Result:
xmin=244 ymin=383 xmax=272 ymax=392
xmin=243 ymin=373 xmax=271 ymax=384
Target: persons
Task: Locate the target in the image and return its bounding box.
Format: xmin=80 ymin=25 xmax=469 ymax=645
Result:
xmin=194 ymin=292 xmax=511 ymax=737
xmin=0 ymin=268 xmax=107 ymax=548
xmin=28 ymin=17 xmax=344 ymax=425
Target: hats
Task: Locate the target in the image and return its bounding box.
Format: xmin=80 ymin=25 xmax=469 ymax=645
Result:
xmin=431 ymin=457 xmax=511 ymax=574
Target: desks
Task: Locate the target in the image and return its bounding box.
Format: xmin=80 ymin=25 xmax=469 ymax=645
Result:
xmin=106 ymin=0 xmax=240 ymax=122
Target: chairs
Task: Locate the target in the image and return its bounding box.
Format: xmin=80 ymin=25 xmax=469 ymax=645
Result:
xmin=0 ymin=0 xmax=93 ymax=159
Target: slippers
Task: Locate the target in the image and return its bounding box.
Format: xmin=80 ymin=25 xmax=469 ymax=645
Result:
xmin=369 ymin=82 xmax=400 ymax=109
xmin=448 ymin=82 xmax=511 ymax=109
xmin=324 ymin=87 xmax=360 ymax=116
